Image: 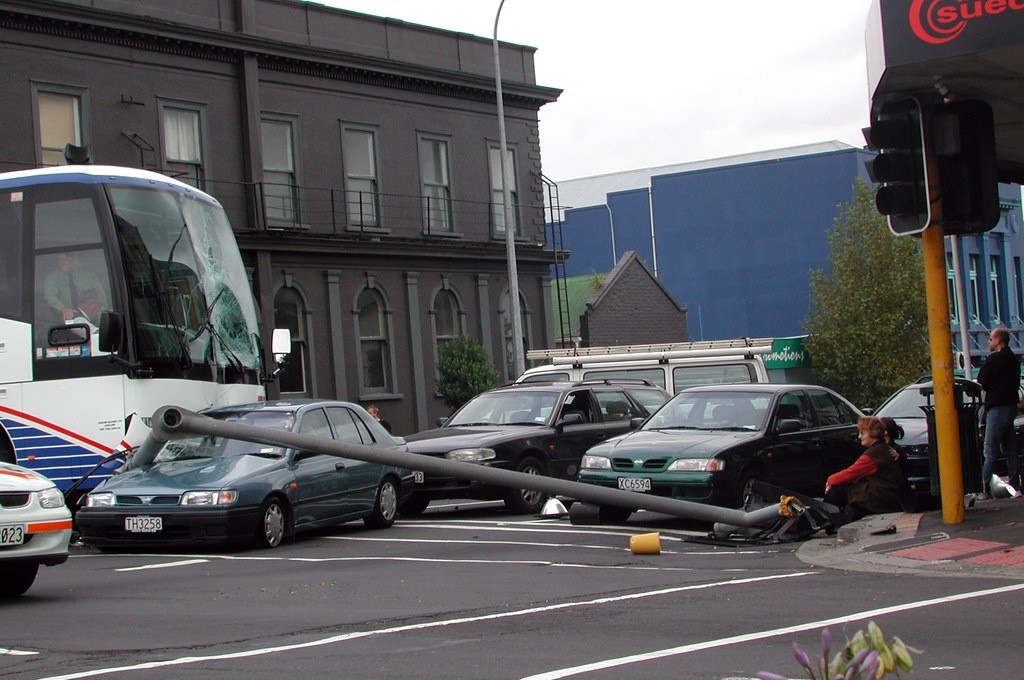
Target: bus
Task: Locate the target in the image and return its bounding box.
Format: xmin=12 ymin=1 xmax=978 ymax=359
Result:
xmin=0 ymin=165 xmax=292 ymax=545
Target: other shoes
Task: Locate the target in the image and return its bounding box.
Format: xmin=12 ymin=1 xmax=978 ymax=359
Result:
xmin=1005 ymin=490 xmax=1022 ymax=499
xmin=978 ymin=492 xmax=992 ymax=500
xmin=818 ymin=527 xmax=839 ymax=537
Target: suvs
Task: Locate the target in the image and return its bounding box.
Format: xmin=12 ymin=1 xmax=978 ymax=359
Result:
xmin=399 ymin=377 xmax=681 ymax=516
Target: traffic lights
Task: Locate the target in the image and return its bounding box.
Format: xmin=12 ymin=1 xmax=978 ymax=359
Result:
xmin=870 ymin=95 xmax=931 ymax=237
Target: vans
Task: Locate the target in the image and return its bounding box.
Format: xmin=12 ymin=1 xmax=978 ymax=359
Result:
xmin=478 ymin=337 xmax=776 ymax=432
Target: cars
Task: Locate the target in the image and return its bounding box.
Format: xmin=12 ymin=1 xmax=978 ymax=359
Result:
xmin=575 ymin=382 xmax=871 ymax=534
xmin=74 ymin=398 xmax=417 ymax=551
xmin=0 ymin=460 xmax=73 ymax=604
xmin=862 ymin=374 xmax=1024 ymax=496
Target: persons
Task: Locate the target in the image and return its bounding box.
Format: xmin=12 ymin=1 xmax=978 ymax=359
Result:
xmin=822 ymin=416 xmax=912 ymax=535
xmin=977 ymin=329 xmax=1024 ymax=501
xmin=367 ymin=406 xmax=391 ymax=432
xmin=43 ymin=253 xmax=106 ymax=322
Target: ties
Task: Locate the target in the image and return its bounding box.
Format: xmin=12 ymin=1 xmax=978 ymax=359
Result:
xmin=68 ymin=272 xmax=78 ymax=310
